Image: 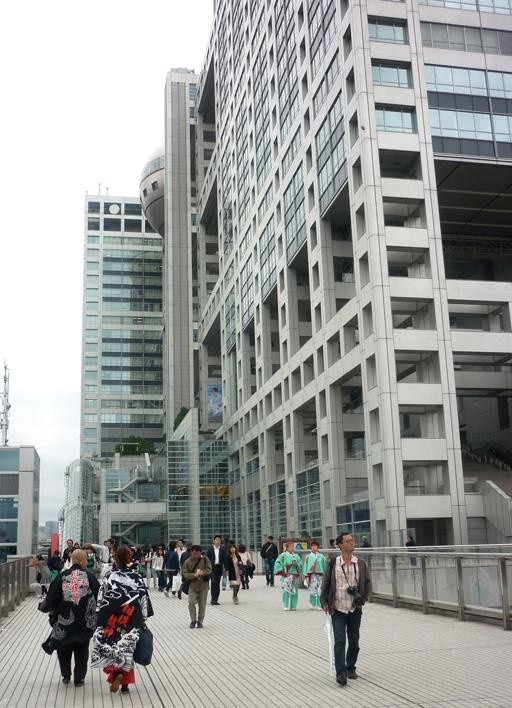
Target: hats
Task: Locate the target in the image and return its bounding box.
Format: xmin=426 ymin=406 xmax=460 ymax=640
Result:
xmin=362 ymin=536 xmax=367 ymax=542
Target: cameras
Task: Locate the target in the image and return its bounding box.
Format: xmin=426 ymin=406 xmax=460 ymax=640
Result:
xmin=348 ymin=586 xmax=363 ymax=604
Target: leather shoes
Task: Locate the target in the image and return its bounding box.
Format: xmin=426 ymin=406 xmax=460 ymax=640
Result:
xmin=211 ymin=601 xmax=220 ymax=605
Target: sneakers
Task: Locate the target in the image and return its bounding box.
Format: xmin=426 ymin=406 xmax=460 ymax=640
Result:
xmin=283 ymin=607 xmax=288 ymax=610
xmin=222 ymin=587 xmax=226 ymax=590
xmin=190 ymin=621 xmax=196 ymax=628
xmin=290 ymin=608 xmax=296 ymax=611
xmin=161 ymin=588 xmax=164 ymax=591
xmin=164 ymin=589 xmax=169 ymax=597
xmin=198 ymin=624 xmax=202 ymax=627
xmin=41 ymin=597 xmax=46 ymax=602
xmin=122 ymin=687 xmax=129 ymax=694
xmin=74 ymin=678 xmax=85 ymax=688
xmin=234 ymin=597 xmax=239 ymax=604
xmin=310 ymin=605 xmax=314 ymax=610
xmin=347 ymin=670 xmax=357 ymax=678
xmin=63 ymin=672 xmax=72 ymax=684
xmin=147 ymin=586 xmax=150 ymax=589
xmin=38 ymin=595 xmax=44 ymax=598
xmin=158 ymin=588 xmax=161 ymax=592
xmin=110 ymin=671 xmax=123 ymax=692
xmin=247 ymin=586 xmax=249 ymax=589
xmin=318 ymin=607 xmax=322 ymax=610
xmin=242 ymin=587 xmax=245 ymax=590
xmin=336 ymin=672 xmax=347 ymax=684
xmin=154 ymin=586 xmax=157 ymax=588
xmin=172 ymin=594 xmax=176 ymax=597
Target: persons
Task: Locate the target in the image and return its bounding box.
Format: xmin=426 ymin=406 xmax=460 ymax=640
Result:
xmin=88 ymin=546 xmax=154 ymax=694
xmin=229 ymin=543 xmax=242 ymax=606
xmin=75 ymin=540 xmax=83 ymax=551
xmin=155 ymin=548 xmax=166 ymax=591
xmin=304 ymin=542 xmax=328 ymax=611
xmin=48 ymin=550 xmax=65 ymax=576
xmin=183 ymin=546 xmax=212 ymax=630
xmin=177 ymin=543 xmax=192 ymax=600
xmin=321 ymin=531 xmax=371 ymax=684
xmin=85 ymin=546 xmax=98 ymax=574
xmin=167 ymin=543 xmax=177 ymax=595
xmin=105 ymin=539 xmax=117 ymax=567
xmin=260 ymin=536 xmax=277 ymax=586
xmin=38 ymin=548 xmax=102 ymax=687
xmin=329 ymin=536 xmax=338 ymax=550
xmin=26 ymin=553 xmax=54 ymax=599
xmin=360 ymin=535 xmax=374 ymax=549
xmin=239 ymin=544 xmax=255 ymax=590
xmin=274 ymin=544 xmax=303 ymax=611
xmin=221 ymin=535 xmax=230 ymax=594
xmin=176 ymin=539 xmax=191 ymax=591
xmin=63 ymin=540 xmax=75 ymax=558
xmin=206 ymin=535 xmax=229 ymax=605
xmin=158 ymin=544 xmax=172 ymax=588
xmin=145 ymin=544 xmax=158 ymax=588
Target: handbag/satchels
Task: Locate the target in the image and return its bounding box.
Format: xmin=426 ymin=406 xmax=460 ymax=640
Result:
xmin=181 ymin=555 xmax=202 ymax=594
xmin=93 ymin=554 xmax=104 ymax=574
xmin=247 ymin=552 xmax=251 ymax=570
xmin=204 ymin=555 xmax=211 ymax=582
xmin=172 ymin=571 xmax=182 ymax=591
xmin=239 ymin=561 xmax=246 ymax=575
xmin=133 ymin=625 xmax=153 ymax=665
